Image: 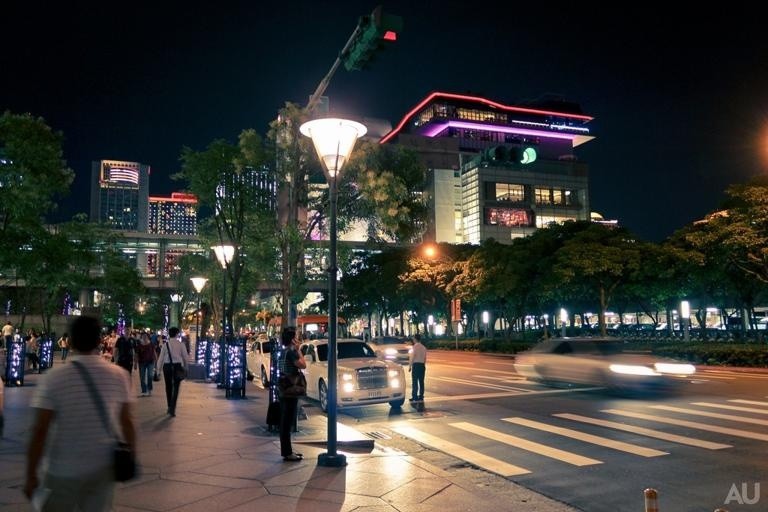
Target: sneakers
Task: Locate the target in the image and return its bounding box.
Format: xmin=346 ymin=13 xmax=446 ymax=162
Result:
xmin=408 ymin=397 xmax=423 ymax=401
xmin=167 ymin=406 xmax=176 ymax=416
xmin=138 ymin=391 xmax=151 ymax=396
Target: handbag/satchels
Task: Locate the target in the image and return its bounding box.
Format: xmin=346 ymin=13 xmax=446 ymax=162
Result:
xmin=113 ymin=440 xmax=135 ymax=482
xmin=175 ymin=367 xmax=186 ymax=381
xmin=153 ymin=370 xmax=160 ymax=381
xmin=279 ymin=370 xmax=307 ymax=398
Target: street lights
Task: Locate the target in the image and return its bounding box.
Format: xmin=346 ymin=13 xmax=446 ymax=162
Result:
xmin=561 ymin=309 xmax=567 ymax=336
xmin=299 ymin=114 xmax=367 ymax=467
xmin=426 ymin=248 xmax=458 ymax=350
xmin=190 ymin=242 xmax=240 ymax=388
xmin=682 ymin=301 xmax=689 ymax=341
xmin=483 ymin=312 xmax=488 ymax=337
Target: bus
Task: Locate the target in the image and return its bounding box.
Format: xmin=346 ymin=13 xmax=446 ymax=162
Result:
xmin=266 ymin=315 xmax=351 ymax=338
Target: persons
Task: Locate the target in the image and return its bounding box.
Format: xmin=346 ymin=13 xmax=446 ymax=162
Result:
xmin=22 ymin=314 xmax=138 ymax=512
xmin=0 ymin=321 xmax=191 ymax=397
xmin=156 ymin=327 xmax=188 ymax=417
xmin=408 ymin=334 xmax=427 ymax=401
xmin=277 ymin=326 xmax=306 ymax=462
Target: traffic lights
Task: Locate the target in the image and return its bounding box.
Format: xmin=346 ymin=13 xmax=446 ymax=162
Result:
xmin=343 ymin=5 xmax=404 ymax=72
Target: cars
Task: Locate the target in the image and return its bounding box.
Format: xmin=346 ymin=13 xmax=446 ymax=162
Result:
xmin=246 ymin=336 xmax=413 ymax=412
xmin=591 ymin=322 xmax=680 ymax=330
xmin=514 ymin=337 xmax=695 ymax=398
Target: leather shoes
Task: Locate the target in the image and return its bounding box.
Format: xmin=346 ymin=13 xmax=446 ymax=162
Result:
xmin=284 ymin=453 xmax=303 ymax=462
